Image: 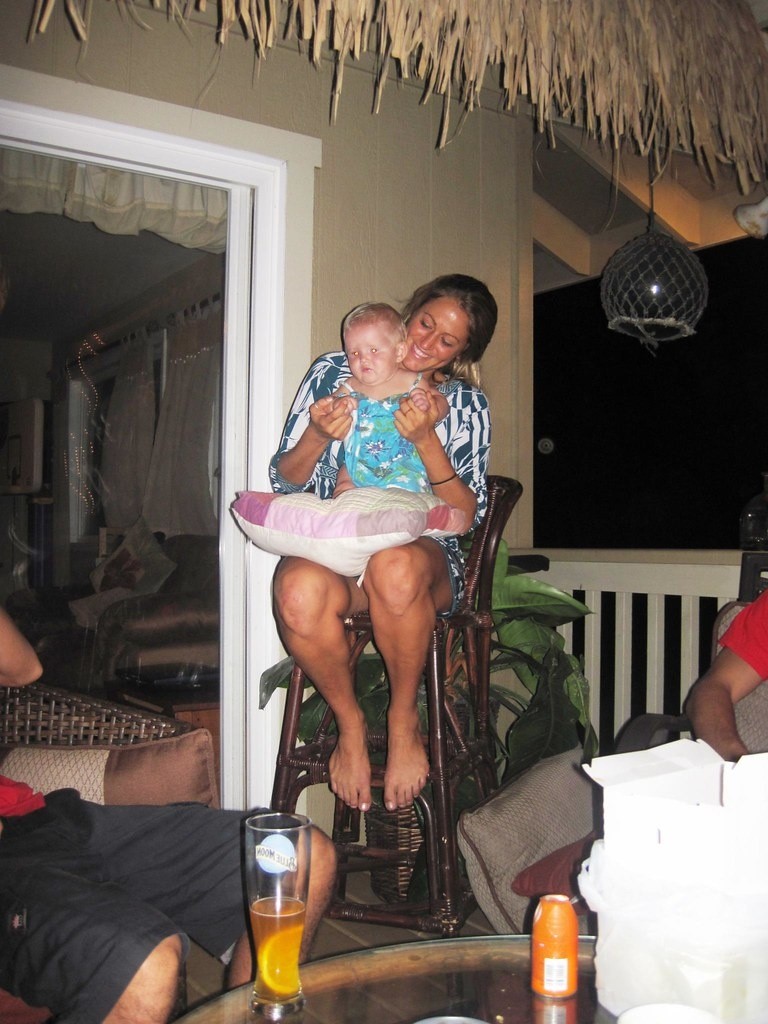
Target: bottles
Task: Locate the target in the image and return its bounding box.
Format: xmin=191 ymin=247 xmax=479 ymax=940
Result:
xmin=530 ymin=894 xmax=579 ymax=999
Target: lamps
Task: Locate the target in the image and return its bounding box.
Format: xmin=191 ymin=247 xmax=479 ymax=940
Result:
xmin=600 ymin=144 xmax=710 ymax=357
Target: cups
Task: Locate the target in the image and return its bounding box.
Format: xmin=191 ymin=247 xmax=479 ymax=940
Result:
xmin=245 ymin=813 xmax=312 ymax=1018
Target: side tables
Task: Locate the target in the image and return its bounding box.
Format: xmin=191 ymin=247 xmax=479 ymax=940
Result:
xmin=115 ymin=686 xmax=221 ymax=803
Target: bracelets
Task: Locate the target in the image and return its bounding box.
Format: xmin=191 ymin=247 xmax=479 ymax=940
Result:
xmin=430 ymin=474 xmax=457 ymax=486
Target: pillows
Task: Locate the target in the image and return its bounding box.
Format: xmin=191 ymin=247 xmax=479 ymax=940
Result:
xmin=457 ymin=741 xmax=594 ymax=937
xmin=69 ymin=586 xmax=138 ymax=632
xmin=234 ymin=490 xmax=467 ymax=579
xmin=89 ymin=515 xmax=178 ymax=596
xmin=0 ymin=728 xmax=221 ymax=810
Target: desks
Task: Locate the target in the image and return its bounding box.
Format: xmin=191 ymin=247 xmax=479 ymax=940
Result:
xmin=171 ymin=935 xmax=618 ymax=1024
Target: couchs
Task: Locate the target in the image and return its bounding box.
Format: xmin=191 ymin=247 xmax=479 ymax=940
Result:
xmin=6 ymin=534 xmax=220 ymax=690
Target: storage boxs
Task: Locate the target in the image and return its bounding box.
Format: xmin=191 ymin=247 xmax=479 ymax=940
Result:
xmin=581 ymin=739 xmax=768 ymax=1024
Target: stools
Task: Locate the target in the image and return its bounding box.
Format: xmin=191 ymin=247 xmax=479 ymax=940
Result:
xmin=272 ymin=475 xmax=524 ymax=938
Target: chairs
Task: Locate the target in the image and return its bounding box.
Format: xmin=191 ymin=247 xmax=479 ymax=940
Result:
xmin=0 ymin=683 xmax=226 ymax=1024
xmin=460 ymin=553 xmax=767 ymax=948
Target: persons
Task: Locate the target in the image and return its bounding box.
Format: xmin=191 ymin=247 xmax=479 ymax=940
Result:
xmin=270 ymin=273 xmax=499 ymax=811
xmin=327 ymin=303 xmax=449 ymax=499
xmin=0 ymin=604 xmax=337 ymax=1024
xmin=685 ymin=589 xmax=768 ymax=765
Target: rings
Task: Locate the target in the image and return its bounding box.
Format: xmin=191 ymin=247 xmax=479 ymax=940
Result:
xmin=314 ymin=404 xmax=318 ymax=409
xmin=405 ymin=409 xmax=412 ymax=416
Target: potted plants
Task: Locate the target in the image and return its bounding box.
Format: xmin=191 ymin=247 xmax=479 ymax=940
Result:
xmin=259 ymin=532 xmax=600 ymax=917
